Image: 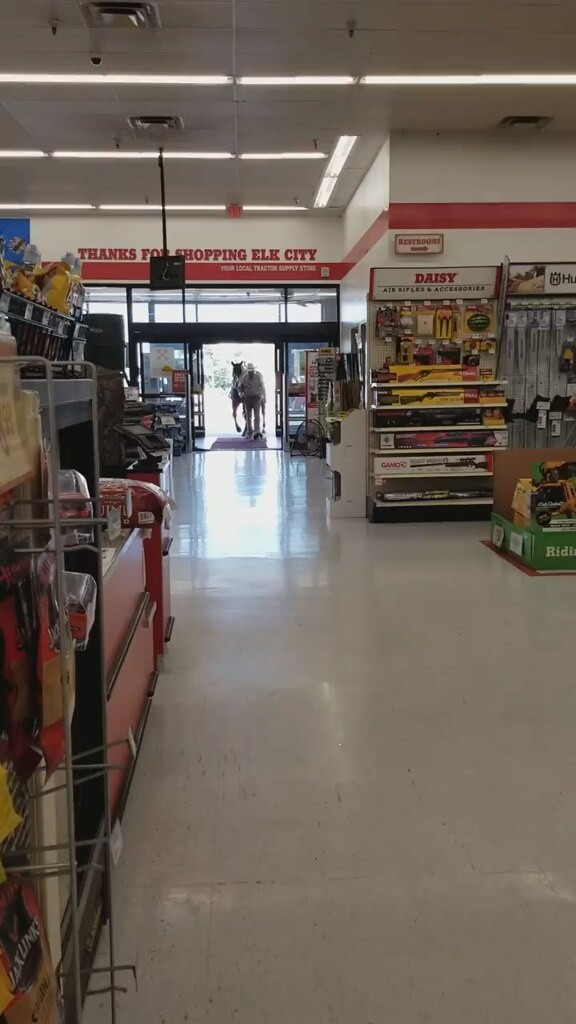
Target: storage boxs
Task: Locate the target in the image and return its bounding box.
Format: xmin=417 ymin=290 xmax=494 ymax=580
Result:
xmin=372 ymin=364 xmax=507 ymax=503
xmin=488 ymin=508 xmax=576 ymax=571
xmin=61 ymin=572 xmax=97 ymax=653
xmin=125 ymin=516 xmax=176 ymax=655
xmin=55 ymin=468 xmax=94 ymax=545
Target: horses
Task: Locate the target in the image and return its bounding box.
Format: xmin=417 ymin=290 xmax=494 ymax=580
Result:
xmin=228 ymin=360 xmax=267 ymax=438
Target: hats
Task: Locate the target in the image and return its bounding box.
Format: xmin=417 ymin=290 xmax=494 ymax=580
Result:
xmin=245 ymin=363 xmax=258 ymax=372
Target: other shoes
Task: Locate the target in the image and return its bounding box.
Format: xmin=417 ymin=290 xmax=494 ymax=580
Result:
xmin=246 ymin=433 xmax=262 ymax=440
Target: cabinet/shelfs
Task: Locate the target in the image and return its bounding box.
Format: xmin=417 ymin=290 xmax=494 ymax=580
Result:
xmin=98 ymin=526 xmax=161 ymax=831
xmin=364 ymin=381 xmax=507 ymax=523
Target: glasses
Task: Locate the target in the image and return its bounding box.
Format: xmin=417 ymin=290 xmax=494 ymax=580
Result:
xmin=316 ymin=360 xmax=334 ymax=415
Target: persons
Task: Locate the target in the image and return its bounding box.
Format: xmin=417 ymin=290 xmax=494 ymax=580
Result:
xmin=238 ymin=363 xmax=266 ymax=440
